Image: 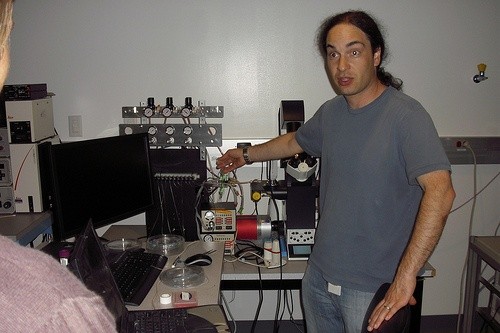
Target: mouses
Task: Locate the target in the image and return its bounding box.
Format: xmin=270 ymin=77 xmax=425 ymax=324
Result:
xmin=184 ymin=254 xmax=212 ymax=266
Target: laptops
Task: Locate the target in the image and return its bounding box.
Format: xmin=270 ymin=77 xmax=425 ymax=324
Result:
xmin=68 ymin=218 xmax=192 ymax=333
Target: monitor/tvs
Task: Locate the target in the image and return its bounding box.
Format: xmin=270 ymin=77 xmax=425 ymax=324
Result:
xmin=38 ymin=132 xmax=155 ymax=242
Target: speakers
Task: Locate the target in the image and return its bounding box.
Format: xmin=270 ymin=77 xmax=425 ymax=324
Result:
xmin=9 ymin=144 xmax=44 ymax=214
xmin=5 ymin=97 xmax=56 ymax=143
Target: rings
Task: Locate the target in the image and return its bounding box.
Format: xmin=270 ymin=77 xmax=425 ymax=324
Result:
xmin=384 ymin=306 xmax=391 ymax=310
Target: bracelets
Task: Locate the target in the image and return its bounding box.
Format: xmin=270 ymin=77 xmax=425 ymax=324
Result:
xmin=243 ymin=146 xmax=252 ymax=165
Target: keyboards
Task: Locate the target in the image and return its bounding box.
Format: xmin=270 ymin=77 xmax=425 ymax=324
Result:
xmin=109 ymin=251 xmax=168 ymax=306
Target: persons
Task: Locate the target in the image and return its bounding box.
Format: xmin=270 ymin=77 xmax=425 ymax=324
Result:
xmin=0 ymin=0 xmax=118 ymax=333
xmin=216 ymin=10 xmax=456 ymax=333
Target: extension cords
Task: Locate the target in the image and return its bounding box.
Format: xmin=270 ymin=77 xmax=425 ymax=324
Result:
xmin=153 ymin=173 xmax=200 ymax=181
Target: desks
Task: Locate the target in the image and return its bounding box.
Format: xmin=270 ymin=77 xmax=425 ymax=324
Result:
xmin=101 ymin=225 xmax=435 ymax=332
xmin=461 ymin=235 xmax=500 ymax=332
xmin=1 ymin=213 xmax=52 ymax=246
